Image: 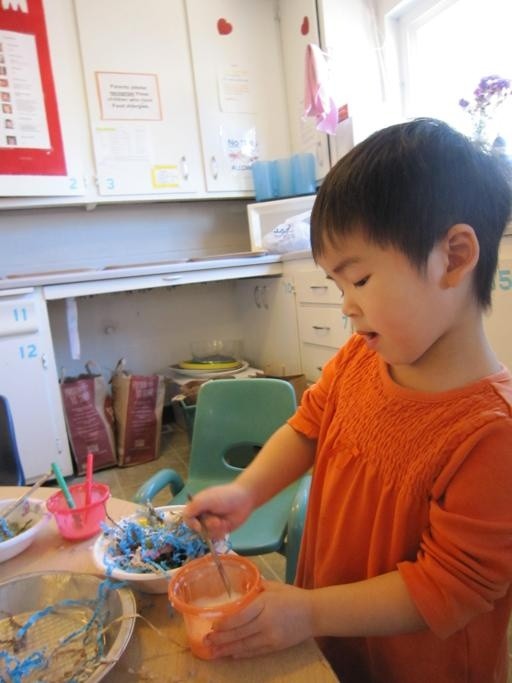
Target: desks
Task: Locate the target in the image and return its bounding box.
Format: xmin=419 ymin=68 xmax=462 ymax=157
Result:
xmin=0 ymin=488 xmax=342 ymax=682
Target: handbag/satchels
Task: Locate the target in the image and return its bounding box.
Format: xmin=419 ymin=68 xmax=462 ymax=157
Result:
xmin=58 ymin=361 xmax=118 ymax=476
xmin=109 ymin=356 xmax=177 ymax=468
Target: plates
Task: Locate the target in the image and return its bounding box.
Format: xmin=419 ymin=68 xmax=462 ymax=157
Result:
xmin=166 ymin=361 xmax=250 ymax=377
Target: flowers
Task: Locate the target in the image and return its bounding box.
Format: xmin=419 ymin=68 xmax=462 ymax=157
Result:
xmin=458 ymin=72 xmax=509 ymax=148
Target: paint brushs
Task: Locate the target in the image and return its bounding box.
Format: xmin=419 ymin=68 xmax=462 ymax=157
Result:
xmin=0 ymin=469 xmax=54 ymax=526
xmin=51 ymin=453 xmax=94 ymax=531
xmin=186 ymin=493 xmax=234 ymax=602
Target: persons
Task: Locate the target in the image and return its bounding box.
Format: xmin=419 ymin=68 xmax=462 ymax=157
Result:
xmin=181 ymin=118 xmax=512 ymax=683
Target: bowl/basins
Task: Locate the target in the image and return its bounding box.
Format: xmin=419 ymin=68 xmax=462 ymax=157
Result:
xmin=1 ymin=571 xmax=137 ymax=682
xmin=92 ymin=505 xmax=229 ymax=595
xmin=0 ymin=484 xmax=111 ymax=565
xmin=188 ymin=340 xmax=240 ymax=364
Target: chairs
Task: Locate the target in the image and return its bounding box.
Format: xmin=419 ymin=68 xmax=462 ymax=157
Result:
xmin=132 ymin=378 xmax=310 ymax=587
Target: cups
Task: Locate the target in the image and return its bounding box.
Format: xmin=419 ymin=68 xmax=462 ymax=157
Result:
xmin=250 ymin=153 xmax=317 ymax=201
xmin=168 ymin=554 xmax=262 ymax=661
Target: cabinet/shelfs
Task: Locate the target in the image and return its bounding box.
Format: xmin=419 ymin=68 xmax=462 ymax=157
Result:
xmin=278 ymin=0 xmax=376 ymax=196
xmin=71 ymin=1 xmax=277 ymax=208
xmin=1 ymin=1 xmax=86 ymax=210
xmin=0 ymin=287 xmax=74 ymax=487
xmin=237 ymin=261 xmax=355 ymax=384
